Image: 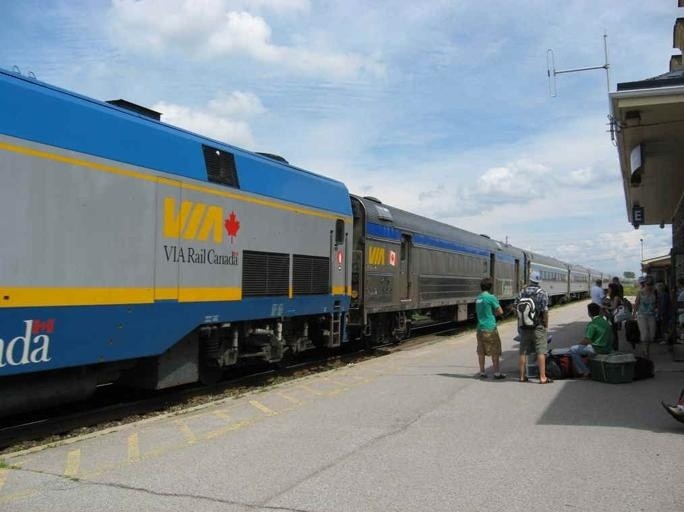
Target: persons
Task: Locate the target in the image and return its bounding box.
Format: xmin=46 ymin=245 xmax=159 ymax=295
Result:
xmin=568 ymin=302 xmax=614 ymax=380
xmin=474 ymin=277 xmax=507 ymax=379
xmin=592 ymin=275 xmax=684 ymax=360
xmin=512 ymin=270 xmax=554 ymax=383
xmin=660 ymin=388 xmax=684 ymax=424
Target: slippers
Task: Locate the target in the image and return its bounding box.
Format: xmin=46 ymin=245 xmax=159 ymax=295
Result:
xmin=541 ymin=378 xmax=553 ymax=384
xmin=519 ymin=376 xmax=528 ymax=382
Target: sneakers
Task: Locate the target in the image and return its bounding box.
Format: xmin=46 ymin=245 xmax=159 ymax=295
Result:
xmin=495 ymin=373 xmax=506 ymax=379
xmin=480 ymin=372 xmax=487 ymax=378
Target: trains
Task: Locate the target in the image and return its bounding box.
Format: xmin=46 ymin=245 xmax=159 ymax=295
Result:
xmin=0 ymin=65 xmax=613 ymax=413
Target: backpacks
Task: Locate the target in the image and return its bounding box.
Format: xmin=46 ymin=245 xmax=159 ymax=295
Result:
xmin=516 ymin=288 xmax=542 ymax=328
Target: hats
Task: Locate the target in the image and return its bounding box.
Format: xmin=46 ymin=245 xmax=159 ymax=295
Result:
xmin=530 ymin=271 xmax=541 ymax=282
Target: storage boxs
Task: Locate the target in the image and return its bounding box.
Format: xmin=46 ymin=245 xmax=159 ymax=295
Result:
xmin=592 ymin=354 xmax=637 ymax=384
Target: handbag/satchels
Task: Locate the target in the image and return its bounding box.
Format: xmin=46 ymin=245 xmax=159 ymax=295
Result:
xmin=546 ymin=354 xmax=572 ymax=379
xmin=625 ymin=320 xmax=640 ymax=342
xmin=613 ymin=304 xmax=630 ymax=323
